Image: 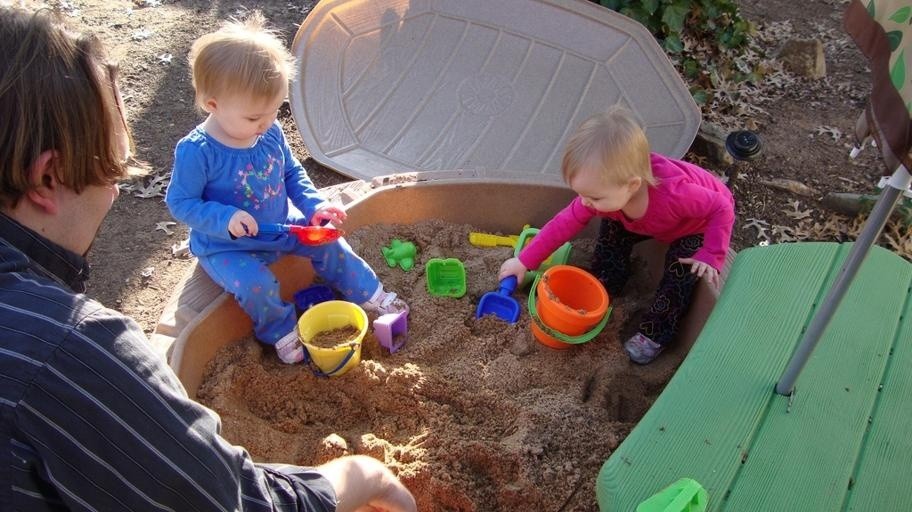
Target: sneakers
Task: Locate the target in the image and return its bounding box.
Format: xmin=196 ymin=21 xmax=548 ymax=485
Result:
xmin=275 ymin=324 xmax=304 ymax=364
xmin=359 ymin=281 xmax=410 ymax=317
xmin=623 ymin=332 xmax=666 ymax=365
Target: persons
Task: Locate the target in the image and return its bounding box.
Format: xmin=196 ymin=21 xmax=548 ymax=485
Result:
xmin=166 ymin=8 xmax=409 ymax=364
xmin=0 ymin=6 xmax=417 ymax=512
xmin=499 ymin=104 xmax=734 ymax=365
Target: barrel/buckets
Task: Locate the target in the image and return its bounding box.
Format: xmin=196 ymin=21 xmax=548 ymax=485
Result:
xmin=530 ymin=265 xmax=610 ymax=348
xmin=298 ymin=300 xmax=369 ymax=378
